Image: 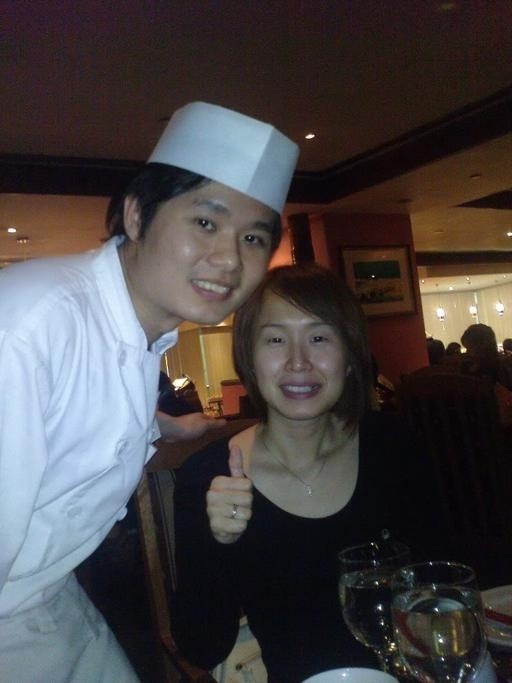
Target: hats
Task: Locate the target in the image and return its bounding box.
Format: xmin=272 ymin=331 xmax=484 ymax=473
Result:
xmin=146 ymin=101 xmax=300 ymax=215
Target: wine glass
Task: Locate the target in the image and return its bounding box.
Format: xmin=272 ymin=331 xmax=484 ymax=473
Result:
xmin=333 ymin=540 xmax=412 ymax=672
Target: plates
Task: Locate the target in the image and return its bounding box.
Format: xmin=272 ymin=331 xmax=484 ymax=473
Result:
xmin=477 ymin=583 xmax=512 ymax=650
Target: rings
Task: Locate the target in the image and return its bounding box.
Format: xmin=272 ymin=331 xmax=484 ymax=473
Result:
xmin=229 ymin=503 xmax=240 ymax=518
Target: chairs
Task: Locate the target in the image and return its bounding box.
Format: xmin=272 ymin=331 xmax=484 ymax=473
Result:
xmin=132 ymin=416 xmax=264 ymax=682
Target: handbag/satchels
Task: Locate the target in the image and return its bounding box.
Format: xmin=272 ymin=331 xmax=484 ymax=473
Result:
xmin=212 ymin=615 xmax=268 ymax=683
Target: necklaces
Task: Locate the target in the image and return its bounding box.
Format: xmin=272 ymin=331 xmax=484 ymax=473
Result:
xmin=257 ymin=428 xmax=335 ymax=499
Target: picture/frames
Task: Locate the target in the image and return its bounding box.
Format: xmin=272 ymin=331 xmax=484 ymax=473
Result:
xmin=337 ymin=243 xmax=421 ymax=320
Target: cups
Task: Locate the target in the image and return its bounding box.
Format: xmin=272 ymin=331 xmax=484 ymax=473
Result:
xmin=389 ymin=559 xmax=486 ymax=683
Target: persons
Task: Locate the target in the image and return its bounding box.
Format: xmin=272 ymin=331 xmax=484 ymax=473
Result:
xmin=165 ymin=258 xmax=445 ymax=682
xmin=406 ymin=319 xmax=512 ymax=478
xmin=2 ymin=94 xmax=305 ymax=682
